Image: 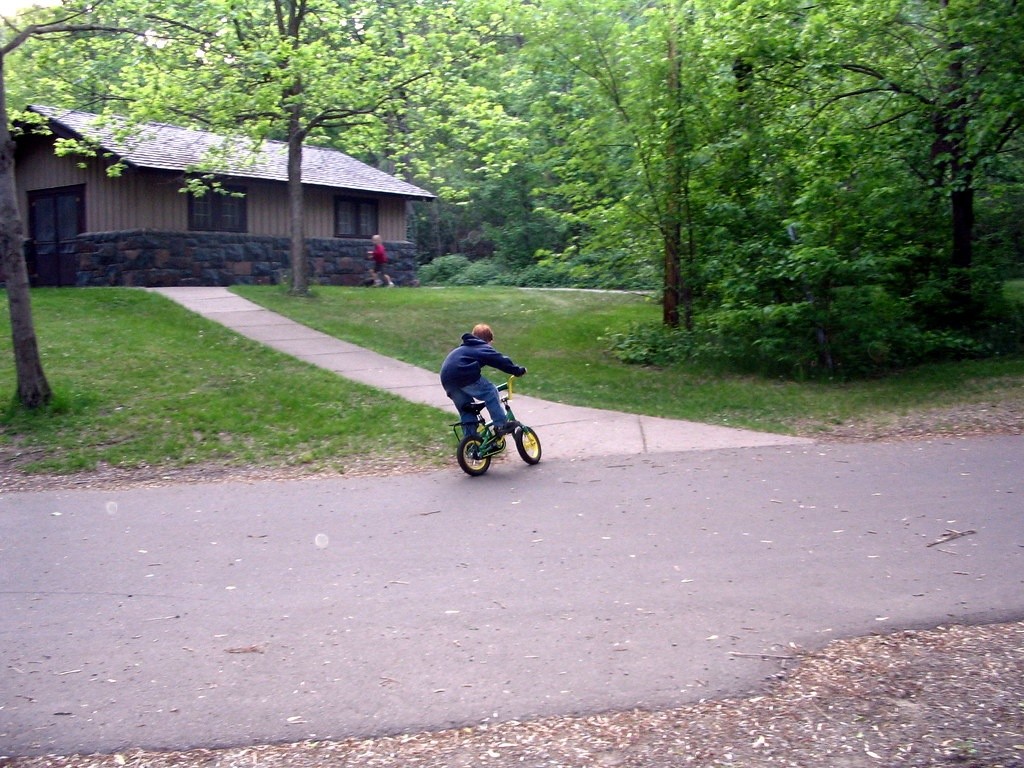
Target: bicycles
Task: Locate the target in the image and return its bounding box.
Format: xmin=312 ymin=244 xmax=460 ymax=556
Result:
xmin=446 ymin=372 xmax=542 ymax=477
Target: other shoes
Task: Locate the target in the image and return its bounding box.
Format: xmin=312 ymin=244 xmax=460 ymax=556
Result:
xmin=387 ymin=282 xmax=396 ymax=288
xmin=373 ymin=280 xmax=383 ymax=286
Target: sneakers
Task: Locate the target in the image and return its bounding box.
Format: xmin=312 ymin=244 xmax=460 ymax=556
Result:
xmin=494 ymin=421 xmax=520 ymax=437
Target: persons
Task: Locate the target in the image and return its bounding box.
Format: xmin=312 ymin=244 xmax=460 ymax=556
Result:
xmin=440 ymin=323 xmax=526 ymax=438
xmin=367 ymin=235 xmax=393 ymax=287
